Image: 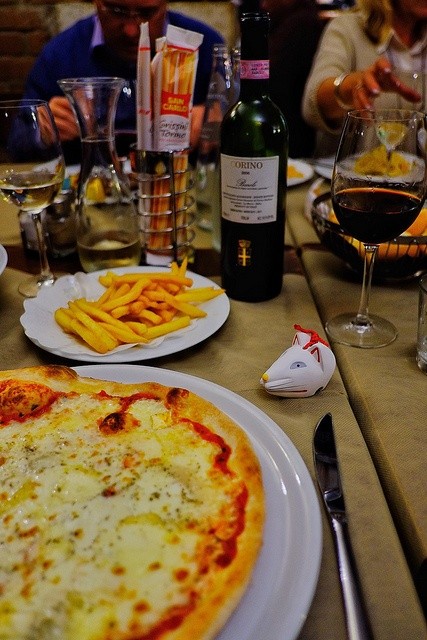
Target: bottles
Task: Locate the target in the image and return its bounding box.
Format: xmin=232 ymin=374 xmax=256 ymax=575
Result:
xmin=59 ymin=84 xmax=141 ymax=272
xmin=220 ymin=12 xmax=289 ymax=303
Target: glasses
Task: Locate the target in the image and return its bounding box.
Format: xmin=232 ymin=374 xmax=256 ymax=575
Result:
xmin=98 ymin=1 xmax=161 ymax=20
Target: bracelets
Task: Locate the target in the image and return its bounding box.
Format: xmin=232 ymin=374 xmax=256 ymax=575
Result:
xmin=330 ymin=68 xmax=365 ymax=114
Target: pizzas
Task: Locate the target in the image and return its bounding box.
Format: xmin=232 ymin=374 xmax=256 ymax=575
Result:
xmin=0 ymin=364 xmax=265 ymax=640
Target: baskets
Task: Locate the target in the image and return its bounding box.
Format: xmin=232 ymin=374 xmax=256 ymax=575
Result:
xmin=311 ymin=190 xmax=427 ymax=278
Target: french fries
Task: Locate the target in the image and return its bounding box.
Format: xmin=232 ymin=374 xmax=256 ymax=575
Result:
xmin=53 ymin=256 xmax=226 ymax=354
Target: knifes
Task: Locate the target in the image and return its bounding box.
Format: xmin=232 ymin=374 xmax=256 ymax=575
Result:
xmin=313 ymin=413 xmax=373 ymax=636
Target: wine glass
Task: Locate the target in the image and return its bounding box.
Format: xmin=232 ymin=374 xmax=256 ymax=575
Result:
xmin=1 ymin=100 xmax=72 ymax=297
xmin=324 ymin=108 xmax=427 ymax=361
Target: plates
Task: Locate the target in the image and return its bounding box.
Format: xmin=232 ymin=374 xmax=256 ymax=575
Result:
xmin=19 ymin=265 xmax=229 ymax=363
xmin=0 ymin=364 xmax=321 ymax=640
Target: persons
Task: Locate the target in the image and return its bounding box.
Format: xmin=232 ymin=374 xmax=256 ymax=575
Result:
xmin=303 ymin=0 xmax=427 ymax=152
xmin=8 ymin=0 xmax=229 ymax=161
